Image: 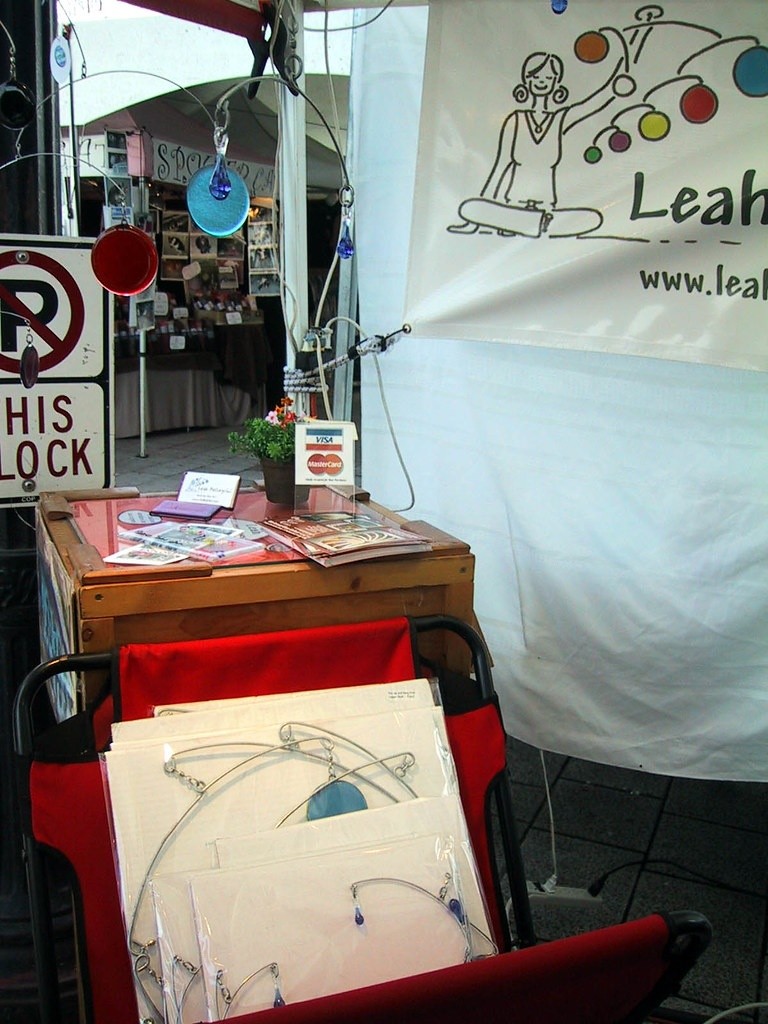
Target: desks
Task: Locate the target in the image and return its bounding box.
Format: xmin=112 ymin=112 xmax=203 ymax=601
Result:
xmin=114 ymin=330 xmax=218 ymax=439
xmin=35 ymin=479 xmax=496 ymax=726
xmin=192 ymin=307 xmax=268 ymax=426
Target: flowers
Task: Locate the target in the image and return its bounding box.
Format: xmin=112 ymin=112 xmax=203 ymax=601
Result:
xmin=227 ymin=397 xmax=316 ymax=461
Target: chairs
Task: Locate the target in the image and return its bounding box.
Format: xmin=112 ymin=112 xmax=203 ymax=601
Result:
xmin=9 ymin=612 xmax=714 ymax=1024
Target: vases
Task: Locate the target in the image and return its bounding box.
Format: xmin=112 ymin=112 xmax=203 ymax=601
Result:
xmin=260 ymin=457 xmax=309 ymax=503
